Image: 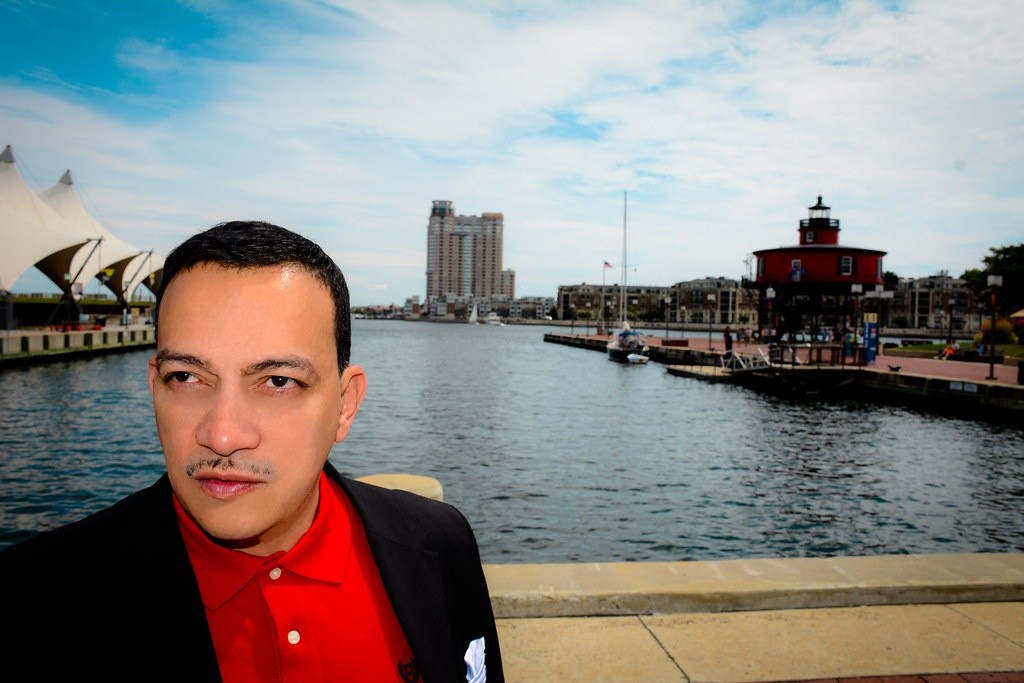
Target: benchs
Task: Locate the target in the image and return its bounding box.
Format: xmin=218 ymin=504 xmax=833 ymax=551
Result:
xmin=965 ymin=349 xmax=1004 ymax=363
xmin=937 ymin=348 xmax=966 ymax=361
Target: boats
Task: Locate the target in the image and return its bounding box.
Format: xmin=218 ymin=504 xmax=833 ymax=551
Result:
xmin=483 ymin=312 xmax=501 ymax=326
xmin=626 ymin=353 xmax=649 ymax=364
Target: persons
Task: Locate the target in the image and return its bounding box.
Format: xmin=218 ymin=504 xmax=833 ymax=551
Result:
xmin=0 ymin=220 xmax=506 ymax=683
xmin=724 ymin=326 xmax=733 ymax=350
xmin=828 ymin=328 xmax=833 ymax=343
xmin=975 ymin=345 xmax=983 ymax=361
xmin=822 ymin=328 xmax=827 ymax=340
xmin=934 ymin=338 xmax=960 ymax=360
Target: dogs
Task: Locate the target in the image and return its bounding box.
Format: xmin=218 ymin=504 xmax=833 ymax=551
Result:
xmin=886 ymin=363 xmax=902 ymax=372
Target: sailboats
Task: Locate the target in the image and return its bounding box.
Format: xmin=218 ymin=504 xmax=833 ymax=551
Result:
xmin=468 ymin=303 xmax=480 ymax=327
xmin=606 ymin=188 xmax=650 ymax=365
